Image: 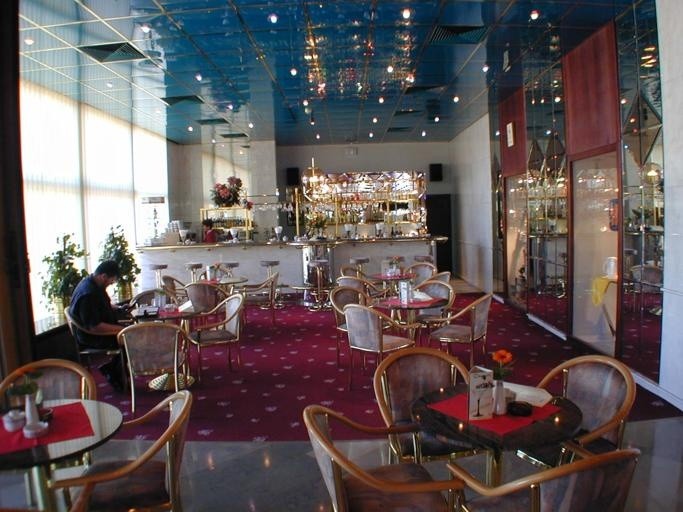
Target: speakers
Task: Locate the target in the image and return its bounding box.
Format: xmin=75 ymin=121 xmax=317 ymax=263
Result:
xmin=429 ymin=163 xmax=443 ymax=182
xmin=286 ymin=167 xmax=299 ymax=186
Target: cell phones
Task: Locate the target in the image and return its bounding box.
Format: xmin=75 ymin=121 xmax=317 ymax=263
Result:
xmin=125 ymin=299 xmax=137 ymax=311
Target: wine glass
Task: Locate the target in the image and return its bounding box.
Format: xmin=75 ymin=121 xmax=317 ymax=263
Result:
xmin=273 ymin=225 xmax=282 ymax=243
xmin=178 ymin=229 xmax=189 ymax=246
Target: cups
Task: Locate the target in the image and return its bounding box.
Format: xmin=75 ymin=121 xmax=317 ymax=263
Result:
xmin=229 ymin=227 xmax=240 ymax=243
xmin=1 ymin=409 xmax=26 ymax=433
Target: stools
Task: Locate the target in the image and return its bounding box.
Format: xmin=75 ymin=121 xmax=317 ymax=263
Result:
xmin=385 ymin=256 xmax=405 ymax=262
xmin=349 ymin=258 xmax=369 ymax=278
xmin=414 ymin=256 xmax=432 ymax=263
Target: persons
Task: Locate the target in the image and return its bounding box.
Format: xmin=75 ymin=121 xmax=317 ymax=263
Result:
xmin=201 ymin=219 xmax=220 ymax=243
xmin=67 ymin=259 xmax=130 ymax=393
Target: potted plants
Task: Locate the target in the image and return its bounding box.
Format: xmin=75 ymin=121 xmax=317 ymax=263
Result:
xmin=98 ymin=224 xmax=141 ymax=299
xmin=40 ymin=232 xmax=90 ymax=314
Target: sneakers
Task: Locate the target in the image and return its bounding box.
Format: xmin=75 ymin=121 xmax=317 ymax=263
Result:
xmin=98 ymin=362 xmax=131 ymax=394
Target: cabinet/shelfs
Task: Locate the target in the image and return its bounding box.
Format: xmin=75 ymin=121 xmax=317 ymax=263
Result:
xmin=303 ymin=178 xmax=421 ymax=225
xmin=199 ymin=208 xmax=254 ymax=243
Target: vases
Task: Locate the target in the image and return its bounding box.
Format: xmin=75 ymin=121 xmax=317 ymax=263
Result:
xmin=219 ymin=202 xmax=232 ymax=207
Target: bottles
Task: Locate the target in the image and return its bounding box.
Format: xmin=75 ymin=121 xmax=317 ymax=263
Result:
xmin=307 ymin=181 xmax=420 ymax=223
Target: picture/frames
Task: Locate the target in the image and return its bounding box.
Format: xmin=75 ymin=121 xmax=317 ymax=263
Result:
xmin=504 ymin=121 xmax=516 ymax=149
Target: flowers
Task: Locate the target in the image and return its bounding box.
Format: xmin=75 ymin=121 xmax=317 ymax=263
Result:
xmin=208 ymin=175 xmax=253 ymax=210
